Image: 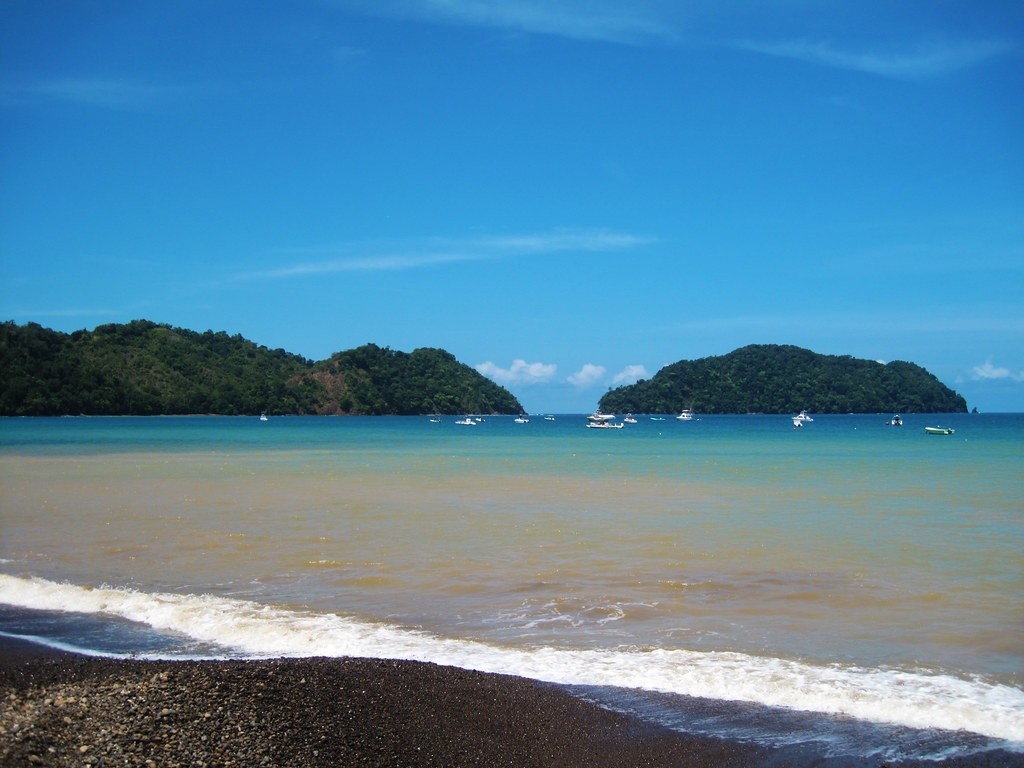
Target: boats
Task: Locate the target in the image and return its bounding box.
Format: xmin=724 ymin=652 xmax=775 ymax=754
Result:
xmin=891 ymin=414 xmax=902 ymax=426
xmin=624 ymin=413 xmax=638 ymax=424
xmin=475 ymin=417 xmax=486 ymax=422
xmin=586 ymin=408 xmax=617 ymax=421
xmin=585 ymin=418 xmax=624 ymax=429
xmin=455 ymin=417 xmax=476 ymax=425
xmin=514 ymin=414 xmax=529 ymax=424
xmin=544 ymin=415 xmax=555 ymax=421
xmin=791 ymin=410 xmax=813 ymax=421
xmin=675 ymin=412 xmax=696 ymax=421
xmin=650 ymin=417 xmax=666 ymax=421
xmin=259 ymin=414 xmax=268 ymax=421
xmin=924 ymin=425 xmax=956 ymax=435
xmin=793 ymin=419 xmax=803 ymax=427
xmin=430 ymin=417 xmax=442 ymax=423
